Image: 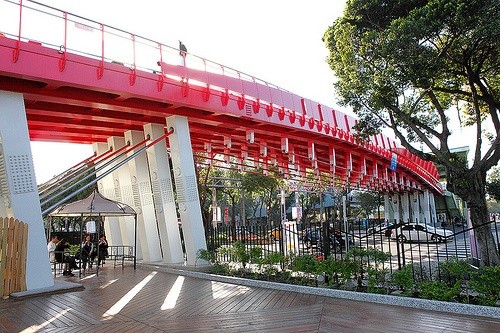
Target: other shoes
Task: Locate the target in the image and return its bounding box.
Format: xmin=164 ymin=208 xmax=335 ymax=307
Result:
xmin=63 ymin=271 xmax=68 ymax=276
xmin=74 ymin=267 xmax=80 ymax=270
xmin=82 ymin=265 xmax=86 ymax=269
xmin=66 ymin=272 xmax=75 ymax=277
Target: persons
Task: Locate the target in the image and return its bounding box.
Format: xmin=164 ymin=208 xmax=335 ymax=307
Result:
xmin=96 ymin=234 xmax=108 ymax=264
xmin=439 ymin=209 xmax=444 ymax=219
xmin=303 ymin=229 xmax=306 ymax=239
xmin=48 ymin=234 xmax=75 ymax=278
xmin=55 ymin=238 xmax=80 ymax=270
xmin=81 ymin=234 xmax=94 ymax=269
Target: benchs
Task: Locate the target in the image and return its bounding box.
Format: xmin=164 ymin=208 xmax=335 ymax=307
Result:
xmin=49 ymin=245 xmax=135 ymax=278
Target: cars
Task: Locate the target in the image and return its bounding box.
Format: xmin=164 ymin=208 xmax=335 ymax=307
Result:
xmin=302 ymin=226 xmax=355 ymax=252
xmin=237 ymin=230 xmax=256 ymax=240
xmin=268 ymin=227 xmax=305 ymax=240
xmin=367 ymin=222 xmax=396 ymax=236
xmin=390 ymin=222 xmax=455 ymax=243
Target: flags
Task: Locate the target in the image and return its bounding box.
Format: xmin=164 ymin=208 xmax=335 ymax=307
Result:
xmin=180 ymin=41 xmax=187 ymax=56
